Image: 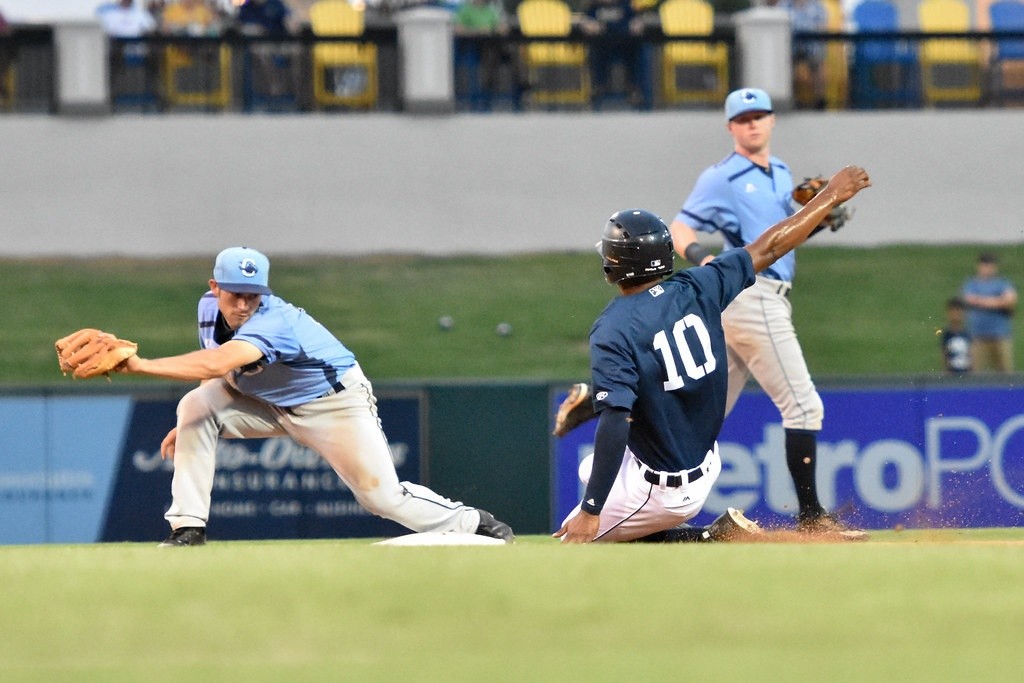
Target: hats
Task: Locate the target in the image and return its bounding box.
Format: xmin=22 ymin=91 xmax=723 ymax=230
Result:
xmin=724 ymin=86 xmax=773 ymax=123
xmin=214 ymin=247 xmax=272 ymax=297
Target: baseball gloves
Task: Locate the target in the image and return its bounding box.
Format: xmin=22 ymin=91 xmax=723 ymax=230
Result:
xmin=55 ymin=329 xmax=138 ymax=379
xmin=791 ymin=178 xmax=848 ymax=232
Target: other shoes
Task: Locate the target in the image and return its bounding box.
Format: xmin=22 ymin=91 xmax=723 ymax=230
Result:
xmin=709 ymin=506 xmax=763 ymax=541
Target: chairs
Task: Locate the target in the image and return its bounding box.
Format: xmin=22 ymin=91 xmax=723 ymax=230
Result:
xmin=0 ymin=0 xmax=1024 ymax=122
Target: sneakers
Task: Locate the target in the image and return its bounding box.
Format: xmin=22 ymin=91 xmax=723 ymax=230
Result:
xmin=158 ymin=523 xmax=206 ymax=548
xmin=796 ymin=511 xmax=870 ymax=538
xmin=555 ymin=382 xmax=593 ymax=436
xmin=475 ymin=508 xmax=513 ymax=543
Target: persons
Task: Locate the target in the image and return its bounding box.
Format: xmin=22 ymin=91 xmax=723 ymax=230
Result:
xmin=938 ymin=296 xmax=978 ymax=375
xmin=552 ymin=164 xmax=872 ymax=545
xmin=96 ymin=0 xmax=830 ymax=114
xmin=54 ymin=247 xmax=514 ymax=546
xmin=668 ymin=86 xmax=870 ymax=543
xmin=961 ymin=253 xmax=1020 ymax=372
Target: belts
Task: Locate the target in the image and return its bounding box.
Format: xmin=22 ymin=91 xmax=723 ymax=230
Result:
xmin=317 ymin=382 xmax=345 ymax=399
xmin=638 ymin=458 xmax=704 ymax=488
xmin=778 ymin=286 xmax=794 ymax=298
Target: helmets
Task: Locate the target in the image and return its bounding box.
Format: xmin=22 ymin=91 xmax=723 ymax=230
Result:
xmin=598 ymin=210 xmax=675 ymax=286
